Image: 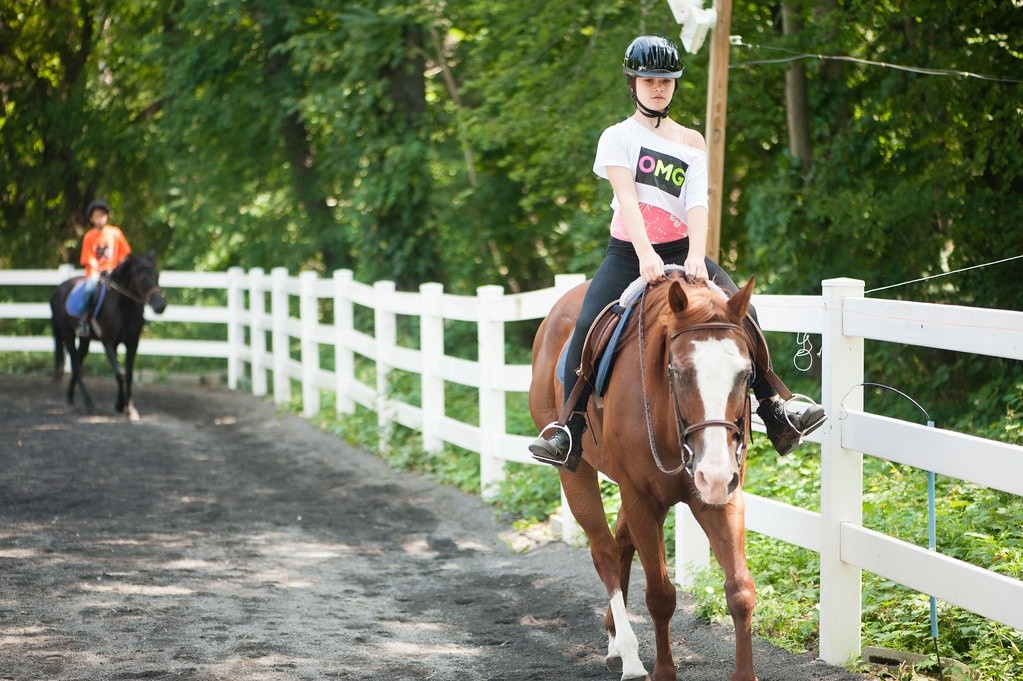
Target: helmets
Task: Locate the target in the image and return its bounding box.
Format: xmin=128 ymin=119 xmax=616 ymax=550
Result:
xmin=622 ymin=34 xmax=685 ymax=77
xmin=86 ymin=200 xmax=110 ymax=216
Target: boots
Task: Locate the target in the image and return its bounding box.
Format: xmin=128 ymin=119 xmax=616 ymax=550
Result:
xmin=528 ymin=413 xmax=589 ymax=473
xmin=756 ymin=396 xmax=824 ymax=456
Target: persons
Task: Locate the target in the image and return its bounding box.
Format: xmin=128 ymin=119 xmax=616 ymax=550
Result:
xmin=529 ymin=33 xmax=824 ymax=471
xmin=73 ymin=200 xmax=134 ymax=337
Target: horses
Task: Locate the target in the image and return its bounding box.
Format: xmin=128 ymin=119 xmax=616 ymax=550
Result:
xmin=528 ymin=271 xmax=763 ymax=681
xmin=48 ymin=248 xmax=166 ymax=421
